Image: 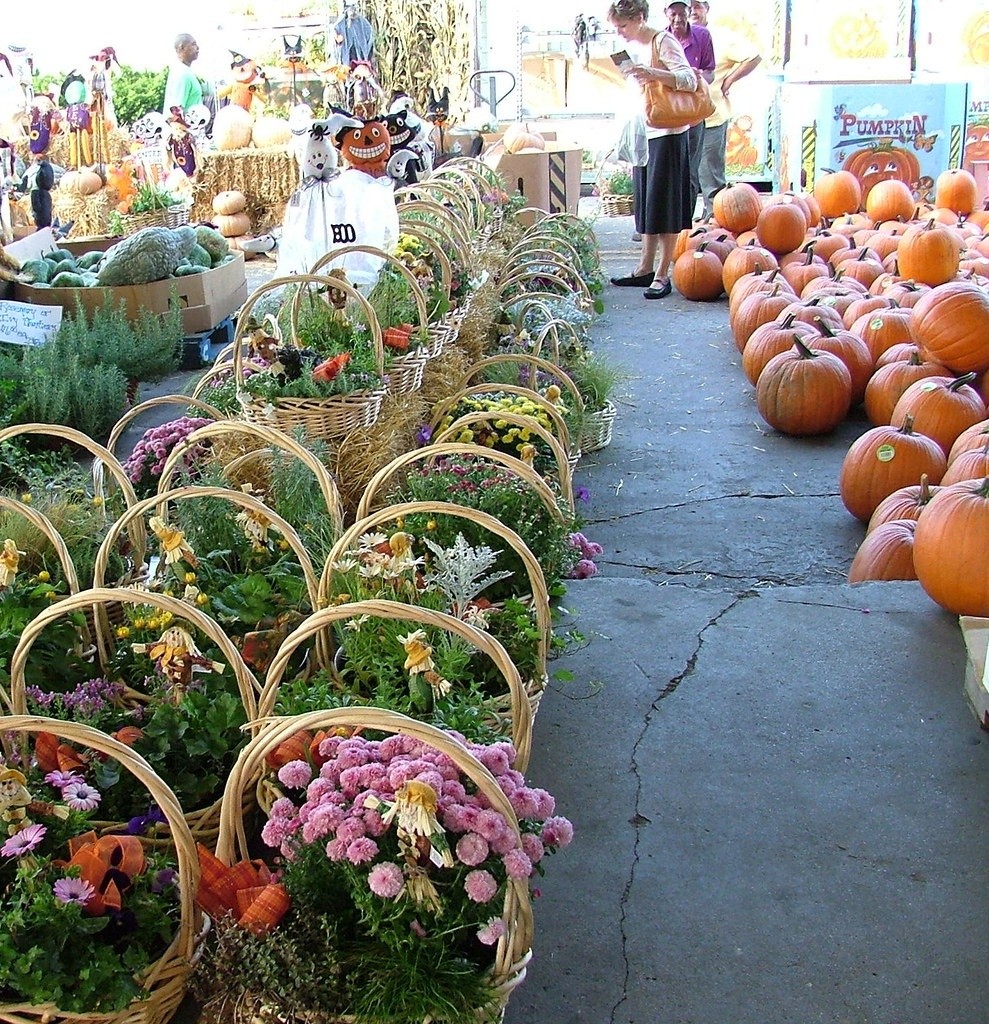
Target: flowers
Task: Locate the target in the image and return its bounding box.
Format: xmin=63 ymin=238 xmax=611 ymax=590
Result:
xmin=0 ymin=168 xmax=619 ymax=1023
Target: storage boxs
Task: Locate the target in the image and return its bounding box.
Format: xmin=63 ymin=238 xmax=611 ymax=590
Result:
xmin=13 ymin=247 xmax=248 ymax=337
xmin=493 ymin=146 xmax=584 ymax=231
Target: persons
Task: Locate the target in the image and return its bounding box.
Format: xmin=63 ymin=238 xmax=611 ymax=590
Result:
xmin=163 ymin=33 xmax=203 ymax=119
xmin=612 ymin=0 xmax=762 ymax=301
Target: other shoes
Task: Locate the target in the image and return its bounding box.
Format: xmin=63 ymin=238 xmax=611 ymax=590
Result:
xmin=643 ymin=278 xmax=671 ymax=298
xmin=610 ymin=267 xmax=655 ymax=287
xmin=633 ymin=231 xmax=643 ymax=241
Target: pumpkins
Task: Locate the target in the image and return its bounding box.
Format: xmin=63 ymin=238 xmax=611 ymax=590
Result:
xmin=672 ymin=166 xmax=989 ymax=617
xmin=22 ymin=103 xmax=293 ymax=288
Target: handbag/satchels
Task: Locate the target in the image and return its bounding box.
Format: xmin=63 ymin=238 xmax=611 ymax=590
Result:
xmin=616 ymin=113 xmax=649 ymax=167
xmin=642 ymin=31 xmax=715 ymax=128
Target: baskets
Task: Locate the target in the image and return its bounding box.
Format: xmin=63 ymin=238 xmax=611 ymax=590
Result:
xmin=1 ymin=155 xmax=616 ymax=1024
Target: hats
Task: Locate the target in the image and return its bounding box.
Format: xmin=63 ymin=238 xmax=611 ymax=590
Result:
xmin=664 ymin=0 xmax=691 ymax=9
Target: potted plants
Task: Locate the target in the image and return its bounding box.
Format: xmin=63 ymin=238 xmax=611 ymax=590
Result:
xmin=1 ymin=281 xmax=189 ymax=478
xmin=109 ymin=185 xmax=188 ymax=237
xmin=595 ymin=167 xmax=636 ymax=216
